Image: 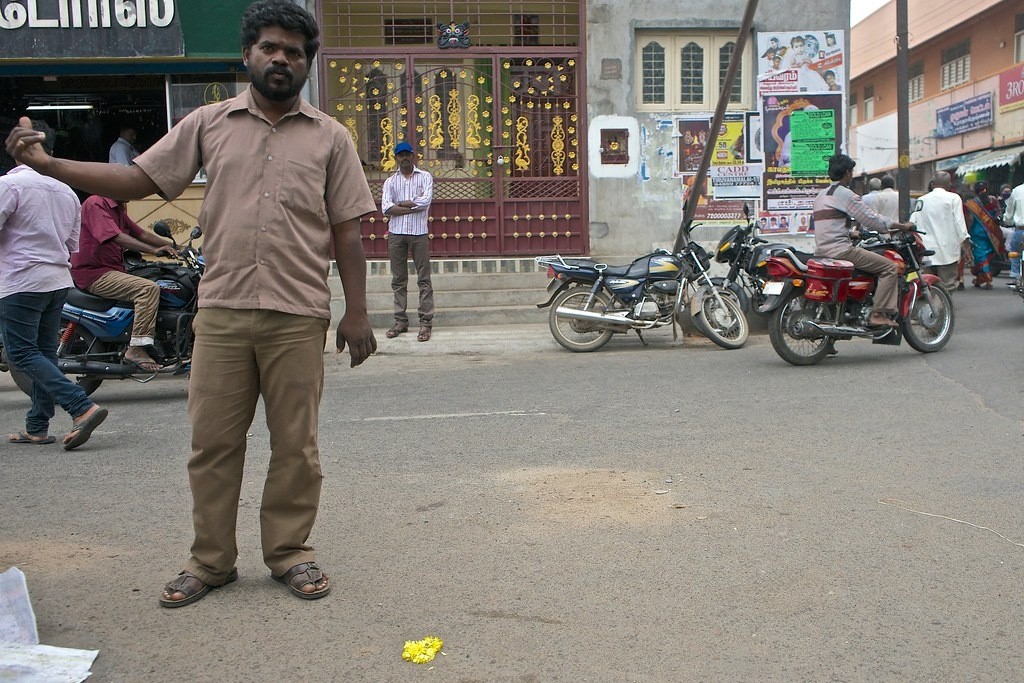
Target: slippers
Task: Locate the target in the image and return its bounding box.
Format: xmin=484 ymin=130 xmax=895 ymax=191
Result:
xmin=271 ymin=561 xmax=331 ymax=600
xmin=160 ymin=567 xmax=239 ymax=608
xmin=124 ymin=353 xmax=157 ymax=373
xmin=64 ymin=408 xmax=108 ymax=451
xmin=8 ymin=431 xmax=56 ymax=445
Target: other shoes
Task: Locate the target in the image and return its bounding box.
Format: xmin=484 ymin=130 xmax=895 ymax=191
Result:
xmin=1006 ymin=281 xmax=1018 ymax=285
xmin=957 ymin=281 xmax=965 ymax=290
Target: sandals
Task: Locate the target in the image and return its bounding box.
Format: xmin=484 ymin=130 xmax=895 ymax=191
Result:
xmin=418 ymin=326 xmax=431 ymax=342
xmin=386 ymin=323 xmax=407 ymax=339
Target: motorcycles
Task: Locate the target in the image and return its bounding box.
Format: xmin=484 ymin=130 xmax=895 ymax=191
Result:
xmin=1 ymin=217 xmax=210 ymax=402
xmin=757 ymin=200 xmax=955 ymax=366
xmin=535 ymin=198 xmax=750 ymax=352
xmin=1002 ymin=223 xmax=1024 ymax=298
xmin=688 ymin=200 xmax=885 ymax=338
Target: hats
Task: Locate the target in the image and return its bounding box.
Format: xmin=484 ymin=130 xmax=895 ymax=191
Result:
xmin=395 ymin=143 xmax=413 ymax=155
xmin=828 ymin=156 xmax=855 ymax=182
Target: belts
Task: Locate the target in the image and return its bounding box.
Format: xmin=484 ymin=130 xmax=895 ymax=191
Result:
xmin=1015 ymin=226 xmax=1024 ymax=230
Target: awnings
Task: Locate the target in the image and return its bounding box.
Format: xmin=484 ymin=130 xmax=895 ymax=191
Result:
xmin=954 ymin=145 xmax=1024 ymax=176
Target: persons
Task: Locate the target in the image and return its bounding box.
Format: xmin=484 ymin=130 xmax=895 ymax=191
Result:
xmin=5 ymin=1 xmax=377 ymax=608
xmin=0 ymin=116 xmax=1024 ymax=450
xmin=762 ymin=34 xmax=842 ymax=92
